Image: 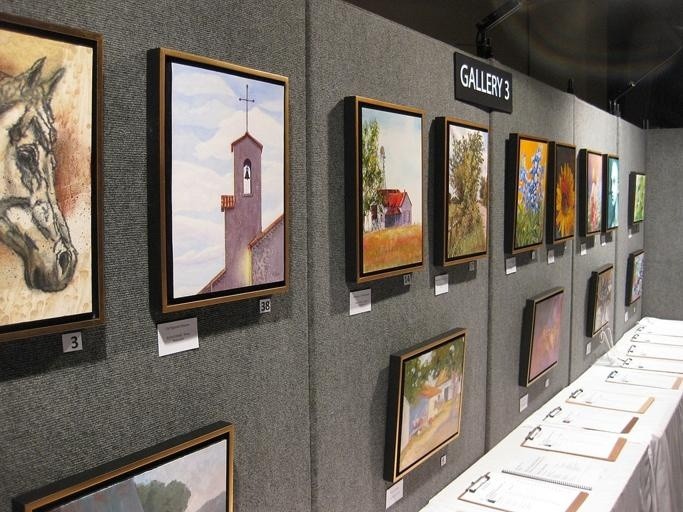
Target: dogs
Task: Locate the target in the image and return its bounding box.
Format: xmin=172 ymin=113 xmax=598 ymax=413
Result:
xmin=0 ymin=55 xmax=78 ymax=294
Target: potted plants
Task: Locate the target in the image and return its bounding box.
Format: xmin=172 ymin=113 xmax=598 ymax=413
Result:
xmin=507 ymin=132 xmax=549 ymax=255
xmin=383 ymin=327 xmax=468 ymax=483
xmin=546 ymin=136 xmax=648 ymax=341
xmin=517 ymin=287 xmax=563 ymax=388
xmin=0 ymin=10 xmax=105 ymax=346
xmin=14 ymin=419 xmax=234 ymax=512
xmin=435 ymin=114 xmax=489 ymax=269
xmin=148 ymin=45 xmax=288 ymax=319
xmin=344 ymin=94 xmax=423 ymax=286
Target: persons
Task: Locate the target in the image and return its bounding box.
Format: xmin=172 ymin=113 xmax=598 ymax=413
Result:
xmin=609 ymin=159 xmax=619 ymax=227
